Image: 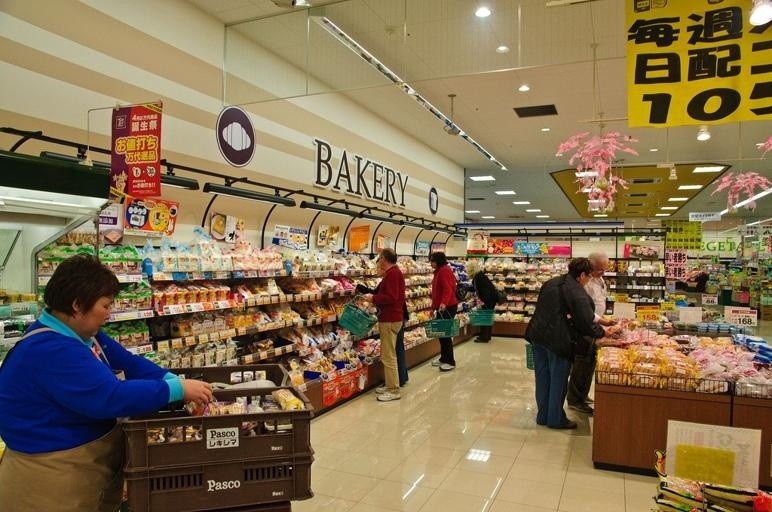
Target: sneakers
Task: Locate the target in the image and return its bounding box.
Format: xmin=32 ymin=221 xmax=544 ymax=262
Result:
xmin=536 ymin=419 xmax=577 ymax=428
xmin=432 ymin=359 xmax=445 ymax=366
xmin=585 ymin=395 xmax=594 ymax=404
xmin=440 ymin=363 xmax=455 ymax=370
xmin=376 ymin=385 xmax=399 ymax=393
xmin=569 ymin=401 xmax=593 ymax=412
xmin=377 ymin=391 xmax=401 ymax=400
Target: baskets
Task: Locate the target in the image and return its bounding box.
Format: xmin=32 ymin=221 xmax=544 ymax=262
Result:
xmin=338 ymin=295 xmax=381 ymax=338
xmin=526 ymin=344 xmax=537 ymax=370
xmin=117 ymin=363 xmax=315 ymax=512
xmin=470 ymin=303 xmax=496 ymax=326
xmin=422 ymin=309 xmax=460 ymax=339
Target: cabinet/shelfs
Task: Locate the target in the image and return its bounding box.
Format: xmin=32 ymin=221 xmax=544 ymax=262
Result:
xmin=605 ymin=256 xmax=667 ymax=309
xmin=593 ymin=371 xmax=772 ymax=493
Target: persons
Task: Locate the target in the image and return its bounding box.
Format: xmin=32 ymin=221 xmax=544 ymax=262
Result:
xmin=525 ymin=257 xmax=613 ymax=429
xmin=0 ymin=252 xmax=213 ymax=512
xmin=566 ymin=250 xmax=618 ymax=413
xmin=363 ymin=248 xmax=405 ymax=402
xmin=352 ymin=259 xmax=409 ymax=386
xmin=466 ymin=258 xmax=500 ymax=342
xmin=428 ymin=252 xmax=459 ymax=372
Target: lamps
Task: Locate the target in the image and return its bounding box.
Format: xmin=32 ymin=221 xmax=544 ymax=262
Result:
xmin=748 ymin=0 xmax=772 ymax=26
xmin=696 ymin=124 xmax=712 ymax=141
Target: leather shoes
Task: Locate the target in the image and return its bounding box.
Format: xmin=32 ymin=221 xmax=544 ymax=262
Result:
xmin=474 ymin=336 xmax=491 ymax=343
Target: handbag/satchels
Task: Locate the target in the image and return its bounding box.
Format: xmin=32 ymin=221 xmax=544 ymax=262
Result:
xmin=456 ymin=285 xmax=467 ymax=301
xmin=497 ymin=293 xmax=506 ymax=305
